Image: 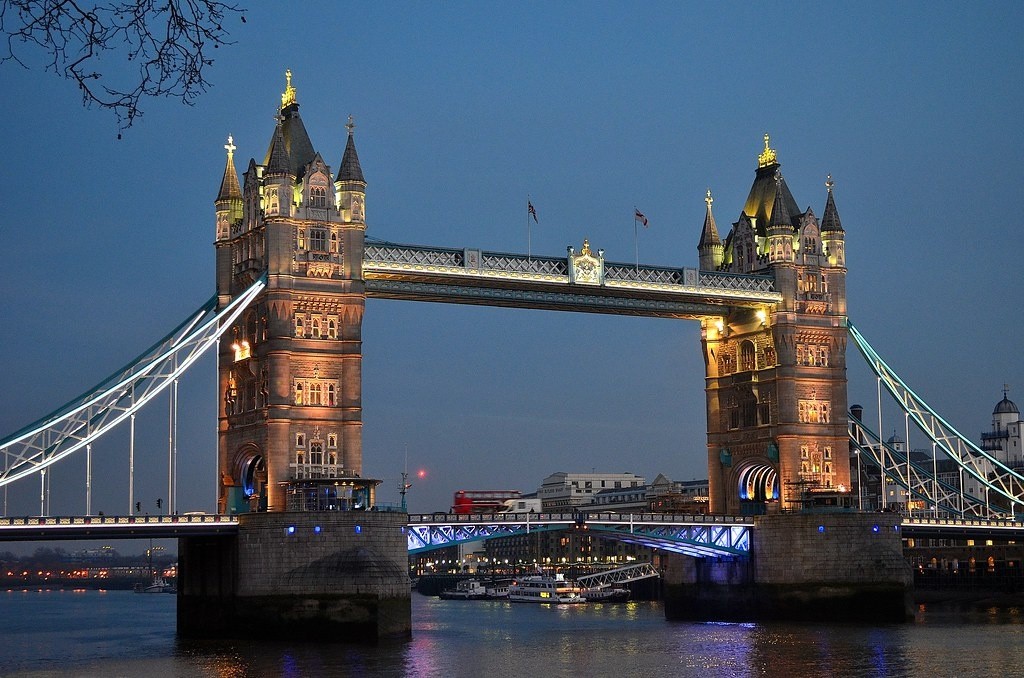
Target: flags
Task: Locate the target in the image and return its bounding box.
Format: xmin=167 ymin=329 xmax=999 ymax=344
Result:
xmin=635 ymin=207 xmax=648 ymax=228
xmin=528 ymin=200 xmax=538 ymax=225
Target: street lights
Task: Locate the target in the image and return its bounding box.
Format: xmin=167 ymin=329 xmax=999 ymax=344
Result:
xmin=478 ymin=559 xmax=480 ymax=572
xmin=485 ymin=558 xmax=488 ymax=568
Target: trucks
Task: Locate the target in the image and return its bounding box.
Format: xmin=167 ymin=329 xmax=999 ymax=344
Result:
xmin=497 ymin=499 xmax=543 ymax=514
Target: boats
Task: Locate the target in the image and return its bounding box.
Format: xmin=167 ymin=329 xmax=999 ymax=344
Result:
xmin=131 ymin=574 xmax=176 ymax=594
xmin=439 ymin=578 xmax=487 ymax=600
xmin=484 ymin=587 xmax=509 ymax=600
xmin=507 ymin=566 xmax=586 ymax=604
xmin=580 ymin=582 xmax=631 ymax=603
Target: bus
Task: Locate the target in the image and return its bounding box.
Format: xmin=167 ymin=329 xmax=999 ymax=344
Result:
xmin=450 ymin=490 xmax=522 ymax=514
